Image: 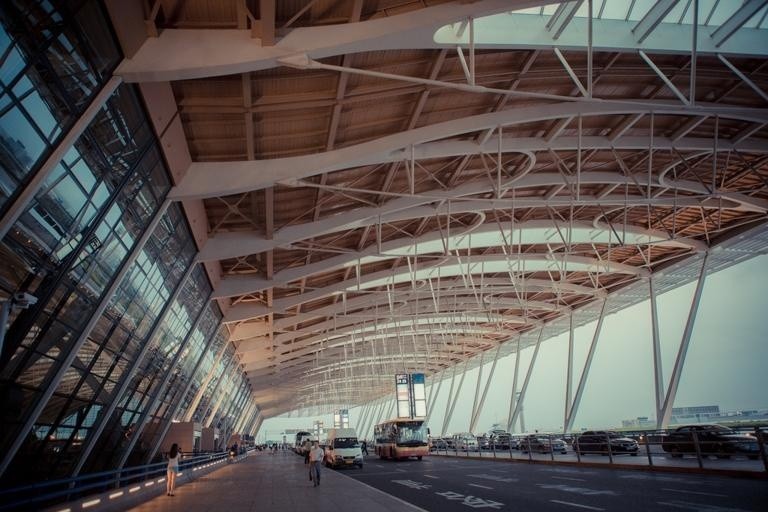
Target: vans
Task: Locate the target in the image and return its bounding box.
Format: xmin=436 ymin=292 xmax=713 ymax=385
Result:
xmin=295 ymin=432 xmax=327 ymax=456
xmin=324 ymin=428 xmax=364 ymax=468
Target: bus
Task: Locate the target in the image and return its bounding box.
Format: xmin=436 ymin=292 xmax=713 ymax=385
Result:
xmin=373 ymin=417 xmax=433 ymax=461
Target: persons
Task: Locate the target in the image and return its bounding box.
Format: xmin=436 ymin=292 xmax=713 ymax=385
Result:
xmin=308 ymin=441 xmax=324 ymax=487
xmin=304 ymin=439 xmax=312 ymax=464
xmin=167 ymin=443 xmax=181 ymax=496
xmin=361 ymin=439 xmax=368 ymax=456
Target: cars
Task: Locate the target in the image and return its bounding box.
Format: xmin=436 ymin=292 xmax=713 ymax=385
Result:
xmin=431 ymin=430 xmax=568 ymax=453
xmin=661 ymin=423 xmax=761 ymax=460
xmin=573 ymin=431 xmax=640 ymax=456
